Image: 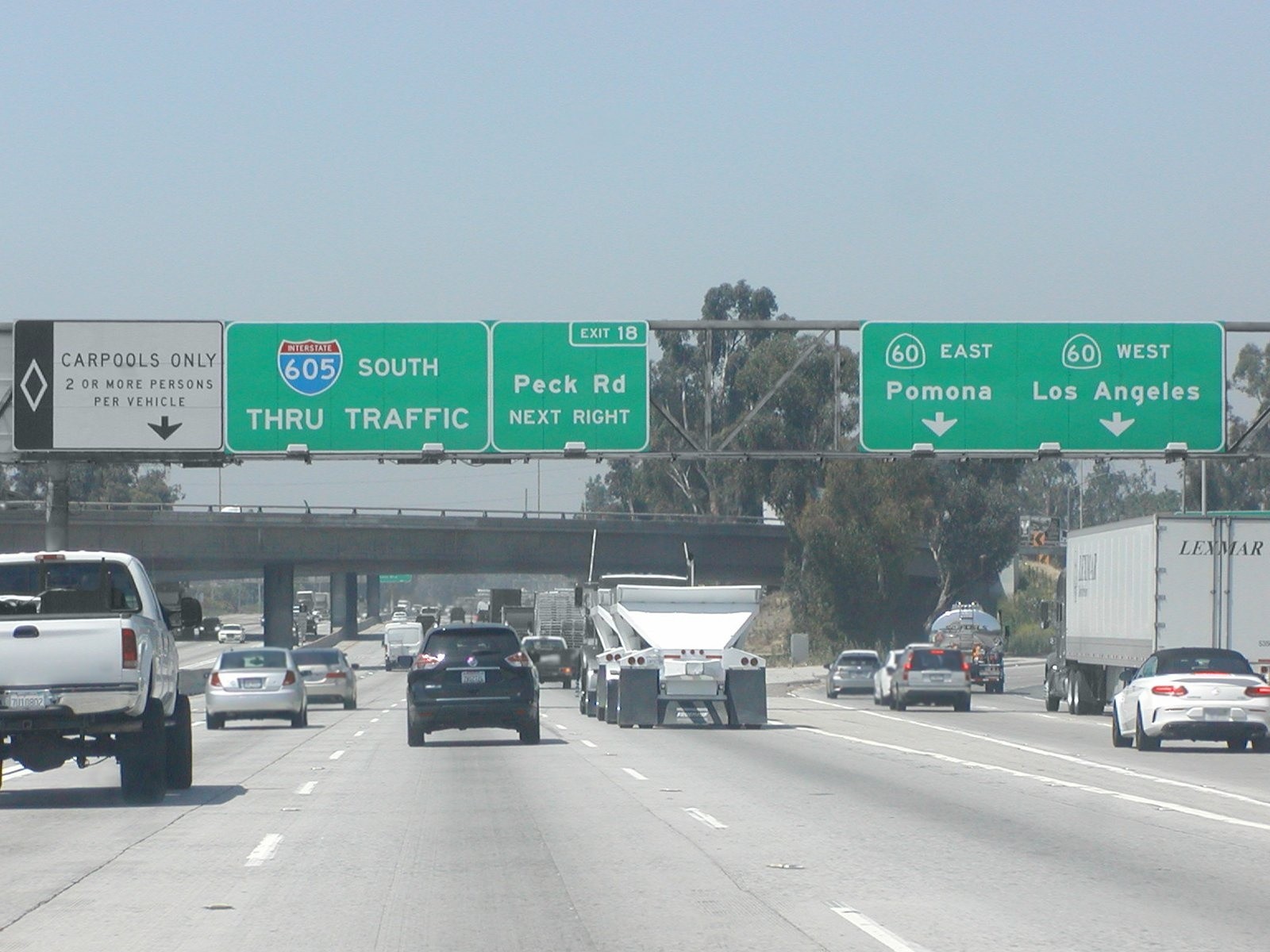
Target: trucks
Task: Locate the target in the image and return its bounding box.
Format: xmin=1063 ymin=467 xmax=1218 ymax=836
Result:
xmin=292 ymin=590 xmax=332 ymax=636
xmin=474 ymin=587 xmax=535 ymax=641
xmin=568 ymin=573 xmax=768 ymax=729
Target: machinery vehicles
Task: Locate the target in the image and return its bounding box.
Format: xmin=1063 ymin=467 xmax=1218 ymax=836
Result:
xmin=925 ymin=601 xmax=1010 ymax=695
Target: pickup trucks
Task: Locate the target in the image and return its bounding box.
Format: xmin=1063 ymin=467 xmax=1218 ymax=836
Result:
xmin=0 ymin=549 xmax=194 ymax=803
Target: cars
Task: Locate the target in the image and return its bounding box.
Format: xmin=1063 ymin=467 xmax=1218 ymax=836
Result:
xmin=391 ymin=599 xmax=466 ymax=635
xmin=823 ymin=650 xmax=882 ymax=700
xmin=217 ymin=623 xmax=246 ymax=644
xmin=291 ymin=647 xmax=360 ymax=710
xmin=202 ymin=646 xmax=313 ymax=730
xmin=870 ymin=648 xmax=907 ymax=707
xmin=1112 ymin=647 xmax=1270 ymax=755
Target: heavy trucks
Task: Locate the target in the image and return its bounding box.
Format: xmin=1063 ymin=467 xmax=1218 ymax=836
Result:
xmin=1038 ymin=508 xmax=1270 ymax=717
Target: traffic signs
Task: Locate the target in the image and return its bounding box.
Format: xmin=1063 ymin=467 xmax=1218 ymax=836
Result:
xmin=858 ymin=319 xmax=1232 ymax=461
xmin=9 ymin=318 xmax=226 ymax=456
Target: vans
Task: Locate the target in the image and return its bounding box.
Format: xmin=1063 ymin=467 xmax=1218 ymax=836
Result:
xmin=381 ymin=622 xmax=424 ymax=672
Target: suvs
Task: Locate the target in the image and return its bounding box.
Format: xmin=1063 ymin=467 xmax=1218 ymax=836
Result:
xmin=522 ymin=636 xmax=574 ymax=689
xmin=397 ymin=621 xmax=542 ymax=747
xmin=198 ymin=617 xmax=223 ymax=638
xmin=886 ymin=642 xmax=972 ymax=711
